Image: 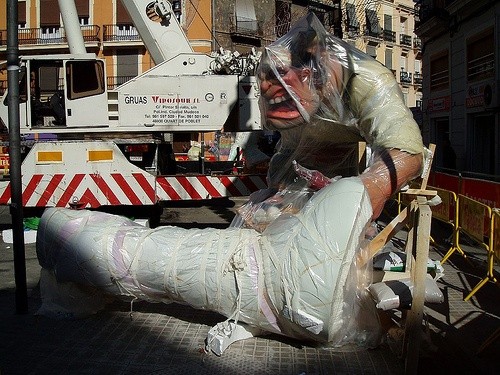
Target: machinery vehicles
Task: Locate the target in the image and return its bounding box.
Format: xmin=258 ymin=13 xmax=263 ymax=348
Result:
xmin=0 ymin=1 xmax=278 ymax=226
